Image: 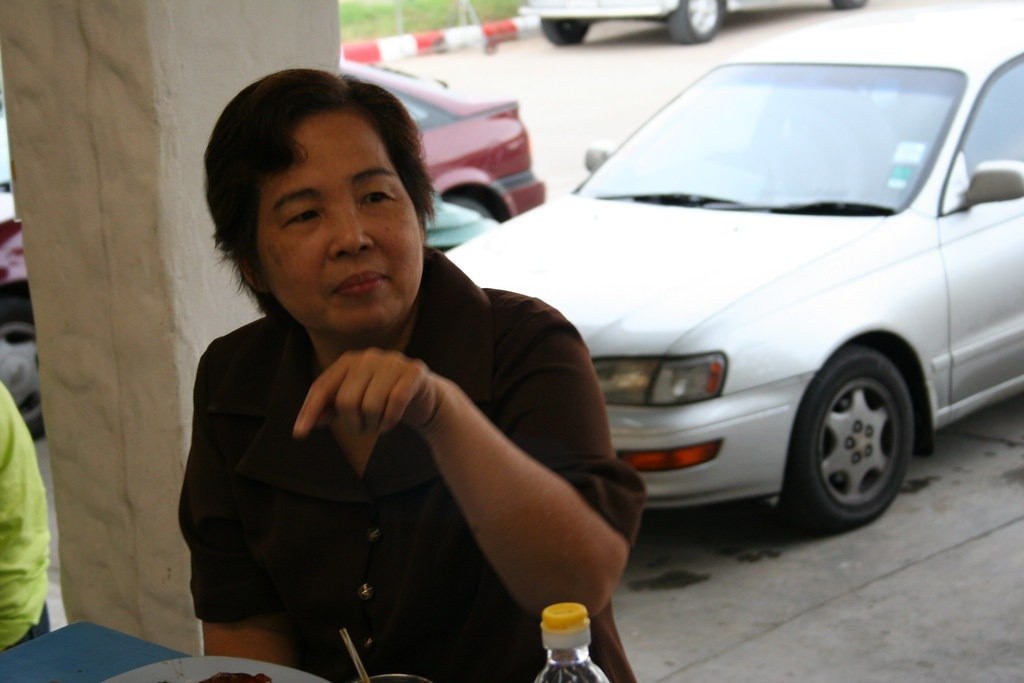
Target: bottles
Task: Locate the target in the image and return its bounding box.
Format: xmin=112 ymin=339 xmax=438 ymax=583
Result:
xmin=534 ymin=603 xmax=611 ymax=683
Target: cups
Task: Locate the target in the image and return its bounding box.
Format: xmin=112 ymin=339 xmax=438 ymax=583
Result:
xmin=354 ymin=673 xmax=433 ymax=683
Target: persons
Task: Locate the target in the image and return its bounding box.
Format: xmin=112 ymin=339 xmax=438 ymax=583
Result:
xmin=177 ymin=69 xmax=648 ymax=683
xmin=0 ymin=381 xmax=51 ymax=653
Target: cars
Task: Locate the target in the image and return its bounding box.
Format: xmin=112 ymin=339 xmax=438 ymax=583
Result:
xmin=530 ymin=0 xmax=866 ymax=46
xmin=1 ymin=56 xmax=548 ymax=442
xmin=447 ymin=0 xmax=1024 ymax=539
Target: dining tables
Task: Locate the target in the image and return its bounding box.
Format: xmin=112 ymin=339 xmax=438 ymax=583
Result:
xmin=0 ymin=621 xmax=192 ymax=683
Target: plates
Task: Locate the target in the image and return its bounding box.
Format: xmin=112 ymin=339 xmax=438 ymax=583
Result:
xmin=100 ymin=655 xmax=330 ymax=683
